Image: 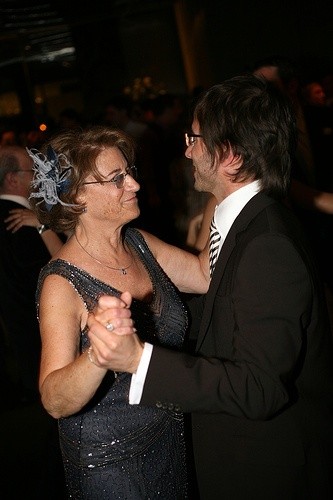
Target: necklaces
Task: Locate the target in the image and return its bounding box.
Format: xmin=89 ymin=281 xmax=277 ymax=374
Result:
xmin=74 ymin=229 xmax=134 ymax=275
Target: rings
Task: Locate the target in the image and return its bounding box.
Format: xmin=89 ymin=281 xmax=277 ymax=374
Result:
xmin=105 ymin=321 xmax=114 ymax=332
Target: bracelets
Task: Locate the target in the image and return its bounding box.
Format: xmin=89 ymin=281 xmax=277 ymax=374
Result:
xmin=87 ymin=345 xmax=95 ymax=363
xmin=36 ymin=224 xmax=51 ymax=235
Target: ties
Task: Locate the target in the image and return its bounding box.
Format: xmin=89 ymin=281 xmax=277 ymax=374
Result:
xmin=208 ymin=215 xmax=221 ymax=283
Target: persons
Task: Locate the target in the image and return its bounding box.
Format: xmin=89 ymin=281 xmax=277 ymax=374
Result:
xmin=0 ymin=56 xmax=333 ymax=500
xmin=86 ymin=76 xmax=333 ymax=500
xmin=27 ymin=126 xmax=210 ymax=500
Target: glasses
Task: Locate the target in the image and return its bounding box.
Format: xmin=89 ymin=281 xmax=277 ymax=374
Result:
xmin=185 ymin=131 xmax=205 ymax=146
xmin=82 ymin=165 xmax=139 ymax=189
